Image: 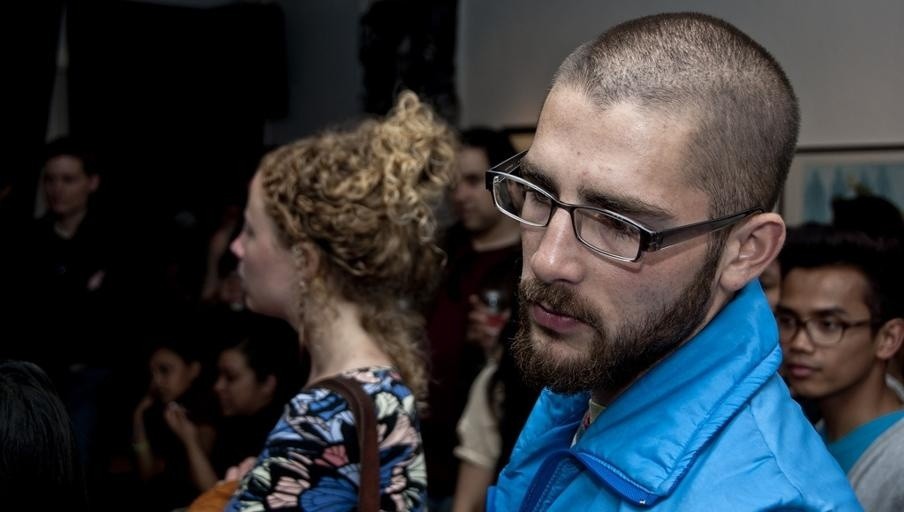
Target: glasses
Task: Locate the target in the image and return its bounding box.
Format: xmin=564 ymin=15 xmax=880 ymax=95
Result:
xmin=483 ymin=147 xmax=761 ymax=265
xmin=772 ymin=308 xmax=880 ymax=348
